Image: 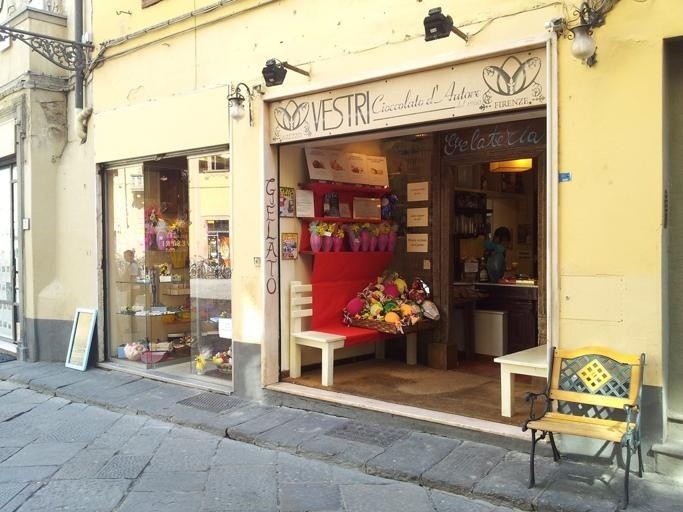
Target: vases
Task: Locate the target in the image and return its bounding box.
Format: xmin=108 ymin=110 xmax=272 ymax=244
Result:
xmin=309 ymin=231 xmax=396 ymax=253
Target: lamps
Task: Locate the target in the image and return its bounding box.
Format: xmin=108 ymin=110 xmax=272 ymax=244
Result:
xmin=227 ymin=82 xmax=253 ymax=127
xmin=566 ymin=3 xmax=601 ymax=68
xmin=490 ymin=158 xmax=532 ymax=172
xmin=261 ymin=57 xmax=310 ymax=87
xmin=423 ymin=6 xmax=468 ymax=43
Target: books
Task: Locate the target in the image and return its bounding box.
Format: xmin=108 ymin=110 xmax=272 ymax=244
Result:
xmin=516 ymin=279 xmax=538 ymax=285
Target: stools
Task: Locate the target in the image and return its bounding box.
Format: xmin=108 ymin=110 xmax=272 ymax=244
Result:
xmin=494 ymin=345 xmax=547 ymax=418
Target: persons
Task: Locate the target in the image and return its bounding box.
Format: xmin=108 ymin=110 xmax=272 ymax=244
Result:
xmin=481 ymin=224 xmax=511 ymax=283
xmin=122 ymin=249 xmax=139 ymax=339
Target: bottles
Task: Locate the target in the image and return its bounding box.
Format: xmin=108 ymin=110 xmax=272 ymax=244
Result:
xmin=478 ymin=257 xmax=489 ymax=283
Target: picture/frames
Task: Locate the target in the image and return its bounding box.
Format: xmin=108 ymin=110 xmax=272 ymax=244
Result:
xmin=353 ymin=197 xmax=382 ymax=220
xmin=65 ymin=308 xmax=97 ymax=372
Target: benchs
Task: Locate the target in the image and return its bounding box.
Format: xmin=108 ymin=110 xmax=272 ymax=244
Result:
xmin=290 ymin=281 xmax=418 ymax=386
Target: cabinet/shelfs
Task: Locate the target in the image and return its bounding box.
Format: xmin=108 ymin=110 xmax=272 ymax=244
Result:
xmin=301 ymin=183 xmax=394 ymax=255
xmin=454 ymin=189 xmax=492 ymax=238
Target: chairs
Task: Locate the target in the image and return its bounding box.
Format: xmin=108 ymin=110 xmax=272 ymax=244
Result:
xmin=523 ymin=347 xmax=645 ymax=509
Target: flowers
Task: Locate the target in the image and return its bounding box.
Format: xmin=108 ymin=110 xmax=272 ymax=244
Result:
xmin=308 ymin=219 xmax=392 ymax=238
